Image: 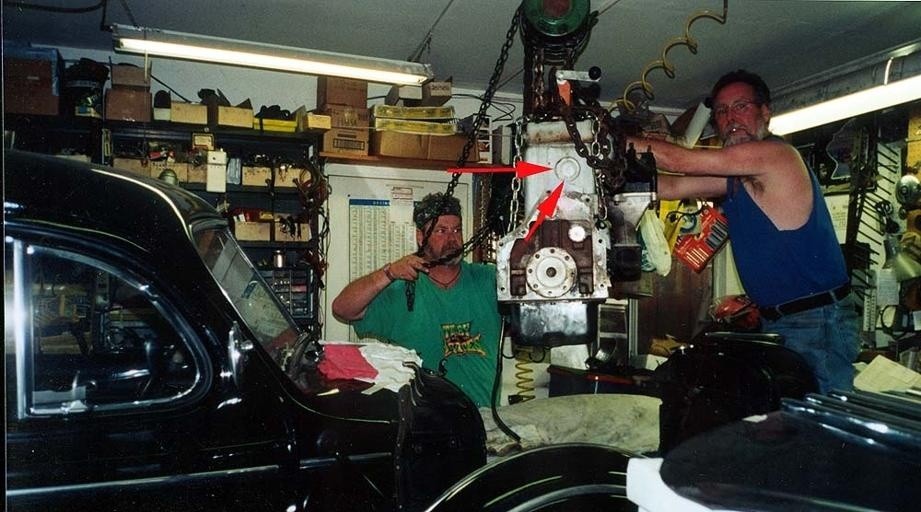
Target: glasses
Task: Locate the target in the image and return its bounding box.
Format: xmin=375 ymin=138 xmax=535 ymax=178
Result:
xmin=715 ymin=97 xmax=760 ymax=121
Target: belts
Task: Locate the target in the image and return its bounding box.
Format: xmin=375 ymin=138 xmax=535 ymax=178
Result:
xmin=759 ymin=280 xmax=852 ymax=325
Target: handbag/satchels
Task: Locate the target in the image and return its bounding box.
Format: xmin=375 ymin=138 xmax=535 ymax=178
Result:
xmin=652 ymin=335 xmax=822 ymax=458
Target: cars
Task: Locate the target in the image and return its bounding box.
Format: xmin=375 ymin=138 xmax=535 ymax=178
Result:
xmin=0 ymin=144 xmax=487 ymax=511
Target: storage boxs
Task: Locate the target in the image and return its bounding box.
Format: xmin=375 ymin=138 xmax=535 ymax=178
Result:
xmin=318 ymin=75 xmax=480 ymax=163
xmin=113 ymin=157 xmax=312 ymax=242
xmin=4 ymin=48 xmax=152 ymax=122
xmin=170 ymin=101 xmax=253 ymax=128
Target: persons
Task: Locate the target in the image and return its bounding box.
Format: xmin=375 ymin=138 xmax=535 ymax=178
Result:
xmin=620 ymin=67 xmax=866 ymax=397
xmin=331 ymin=188 xmax=507 ymax=412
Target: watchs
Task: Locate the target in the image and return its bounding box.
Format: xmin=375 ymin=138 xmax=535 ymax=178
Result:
xmin=383 ymin=262 xmax=397 ymax=283
